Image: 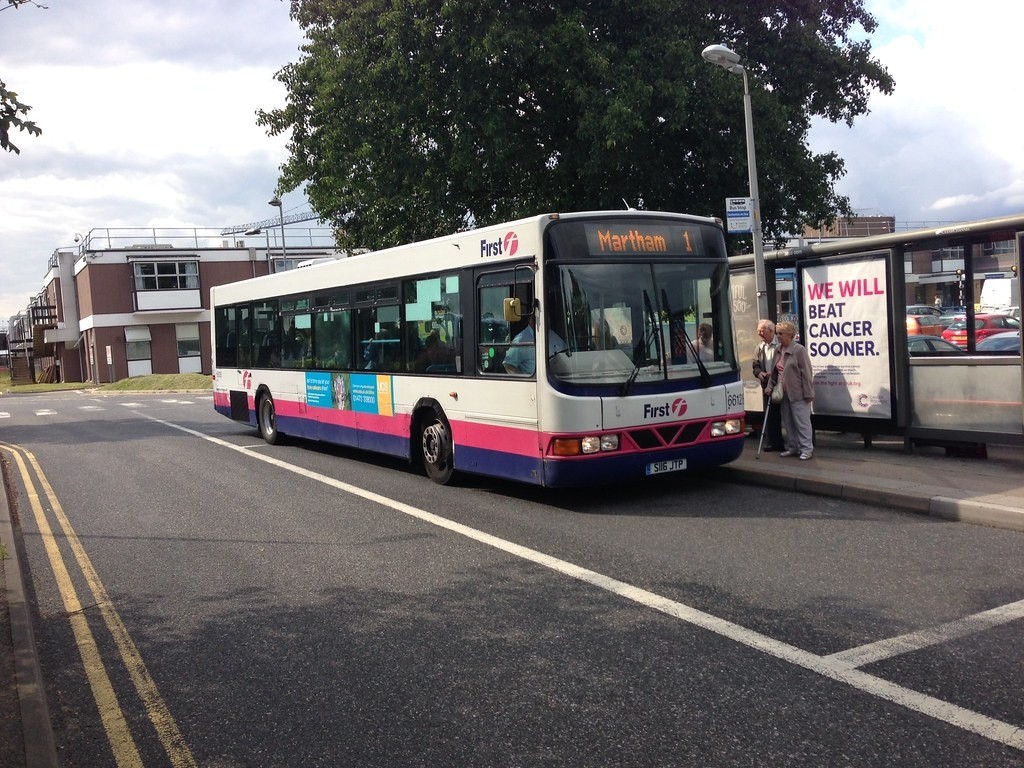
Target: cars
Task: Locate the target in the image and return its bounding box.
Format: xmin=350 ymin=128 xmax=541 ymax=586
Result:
xmin=905 ymin=302 xmax=1023 ymax=357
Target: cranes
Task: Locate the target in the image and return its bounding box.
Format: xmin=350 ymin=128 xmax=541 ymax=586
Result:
xmin=221 ymin=202 xmax=319 ymax=239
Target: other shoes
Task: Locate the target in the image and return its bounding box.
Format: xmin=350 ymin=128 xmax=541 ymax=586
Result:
xmin=800 ymin=453 xmax=812 ymax=460
xmin=764 ymin=444 xmax=785 ymax=452
xmin=780 ymin=450 xmax=801 ymax=457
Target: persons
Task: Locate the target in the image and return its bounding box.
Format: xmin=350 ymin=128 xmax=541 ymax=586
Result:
xmin=239 ymin=317 xmax=262 ymax=346
xmin=502 ymin=311 xmax=568 ymax=374
xmin=593 ymin=319 xmax=617 ymax=350
xmin=262 ymin=319 xmax=305 ymax=347
xmin=686 ymin=324 xmax=714 ymax=362
xmin=414 ymin=330 xmax=445 ymax=373
xmin=752 ymin=319 xmax=785 ymax=451
xmin=935 ymin=295 xmax=942 ymax=307
xmin=765 ymin=321 xmax=815 ymax=459
xmin=363 ymin=329 xmax=397 ymax=370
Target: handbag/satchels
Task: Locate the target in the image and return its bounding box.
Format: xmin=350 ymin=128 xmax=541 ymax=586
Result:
xmin=771 ymin=382 xmax=783 ymax=402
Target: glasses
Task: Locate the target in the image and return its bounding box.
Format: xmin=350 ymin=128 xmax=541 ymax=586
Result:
xmin=775 ymin=332 xmax=787 ymax=335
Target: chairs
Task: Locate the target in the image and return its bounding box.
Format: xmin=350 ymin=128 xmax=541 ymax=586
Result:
xmin=226 ymin=327 xmax=314 ymax=370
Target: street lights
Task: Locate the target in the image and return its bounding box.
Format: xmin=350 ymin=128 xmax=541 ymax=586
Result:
xmin=700 ymin=43 xmax=771 ymax=323
xmin=268 ymin=197 xmax=287 ymax=271
xmin=246 ymin=229 xmax=272 ymax=273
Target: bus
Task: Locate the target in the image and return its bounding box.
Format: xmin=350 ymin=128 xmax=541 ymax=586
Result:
xmin=210 ymin=198 xmax=744 ymax=493
